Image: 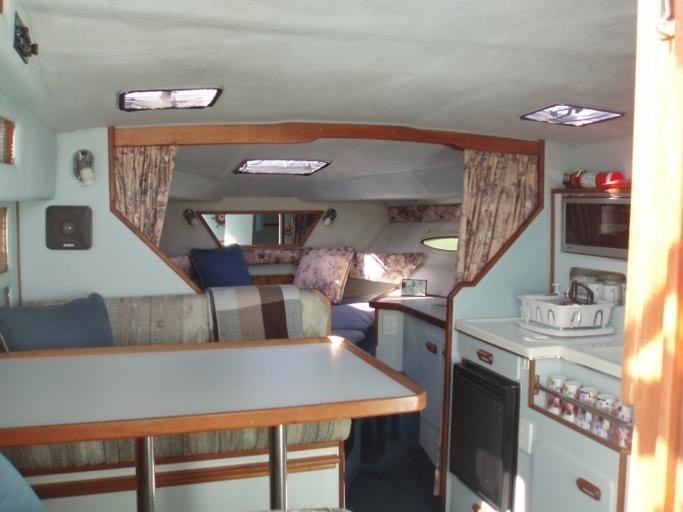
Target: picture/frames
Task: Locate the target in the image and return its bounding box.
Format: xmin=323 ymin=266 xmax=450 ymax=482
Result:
xmin=401 ymin=279 xmax=427 ymax=297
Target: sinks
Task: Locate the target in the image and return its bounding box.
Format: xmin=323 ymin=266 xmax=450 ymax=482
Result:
xmin=518 ymin=293 xmax=614 ymax=329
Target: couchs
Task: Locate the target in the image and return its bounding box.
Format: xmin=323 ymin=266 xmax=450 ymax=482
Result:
xmin=0 ymin=288 xmax=355 ymax=512
xmin=168 ymin=250 xmax=422 ymax=344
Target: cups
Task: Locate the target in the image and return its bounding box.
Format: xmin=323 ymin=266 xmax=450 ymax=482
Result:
xmin=581 ymin=274 xmax=625 ymax=304
xmin=547 ymin=375 xmax=637 ymax=450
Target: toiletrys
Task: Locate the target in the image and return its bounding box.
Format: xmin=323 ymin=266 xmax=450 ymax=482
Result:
xmin=551 ymin=282 xmax=561 ymax=295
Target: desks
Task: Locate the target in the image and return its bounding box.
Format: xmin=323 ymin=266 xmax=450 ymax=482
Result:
xmin=1 ymin=336 xmax=428 ymax=512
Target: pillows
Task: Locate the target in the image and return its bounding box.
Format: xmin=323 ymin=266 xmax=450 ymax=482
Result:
xmin=190 ymin=243 xmax=252 ymax=290
xmin=1 ymin=294 xmax=116 ymax=351
xmin=291 ymin=246 xmax=355 ymax=305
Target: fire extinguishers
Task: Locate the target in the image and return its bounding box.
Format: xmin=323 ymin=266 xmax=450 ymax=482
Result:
xmin=563 ymin=171 xmax=626 ymax=188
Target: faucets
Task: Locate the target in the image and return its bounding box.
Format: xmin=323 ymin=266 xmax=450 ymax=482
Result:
xmin=565 ymin=280 xmax=595 ymax=304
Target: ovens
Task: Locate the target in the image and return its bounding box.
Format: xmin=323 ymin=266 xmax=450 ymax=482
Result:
xmin=448 ymin=357 xmax=523 ymax=512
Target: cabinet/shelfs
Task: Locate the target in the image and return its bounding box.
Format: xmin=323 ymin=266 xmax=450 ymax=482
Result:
xmin=457 ymin=332 xmax=520 ymax=381
xmin=373 ymin=308 xmax=403 ymax=372
xmin=403 ymin=313 xmax=444 ymax=466
xmin=528 ymin=409 xmax=617 ymax=512
